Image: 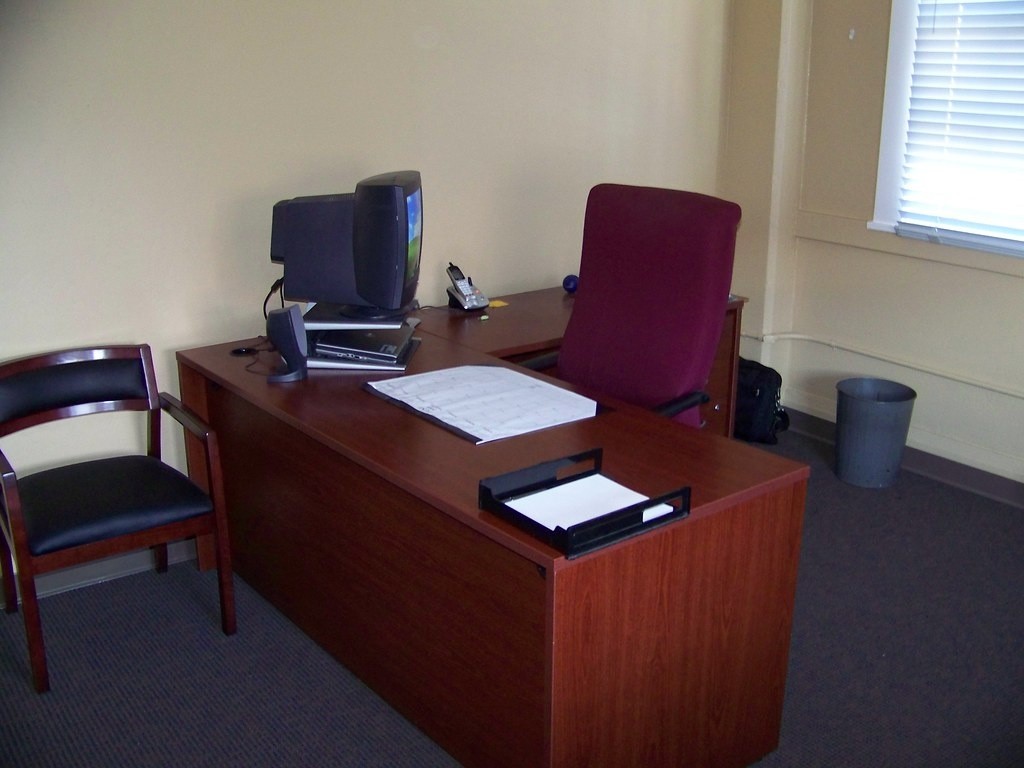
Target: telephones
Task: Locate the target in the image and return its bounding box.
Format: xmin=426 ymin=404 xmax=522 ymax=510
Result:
xmin=445 ymin=261 xmax=490 ymax=311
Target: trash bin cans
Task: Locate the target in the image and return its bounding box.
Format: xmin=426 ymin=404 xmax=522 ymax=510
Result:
xmin=833 ymin=377 xmax=918 ymax=490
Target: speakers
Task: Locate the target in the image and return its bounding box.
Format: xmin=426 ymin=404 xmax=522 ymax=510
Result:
xmin=266 ymin=304 xmax=309 ymax=383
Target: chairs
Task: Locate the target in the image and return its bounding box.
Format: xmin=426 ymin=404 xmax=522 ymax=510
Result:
xmin=0 ymin=344 xmax=236 ymax=694
xmin=518 ymin=183 xmax=742 ymax=429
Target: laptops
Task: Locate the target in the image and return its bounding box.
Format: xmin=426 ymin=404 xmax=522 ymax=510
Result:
xmin=315 ymin=325 xmax=415 ymax=364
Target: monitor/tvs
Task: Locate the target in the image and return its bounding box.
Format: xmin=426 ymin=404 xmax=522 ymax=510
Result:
xmin=269 ymin=170 xmax=424 ymax=321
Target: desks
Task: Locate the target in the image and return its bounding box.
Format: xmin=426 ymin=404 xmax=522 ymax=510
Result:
xmin=175 ymin=330 xmax=811 ymax=768
xmin=404 ymin=285 xmax=749 ymax=440
